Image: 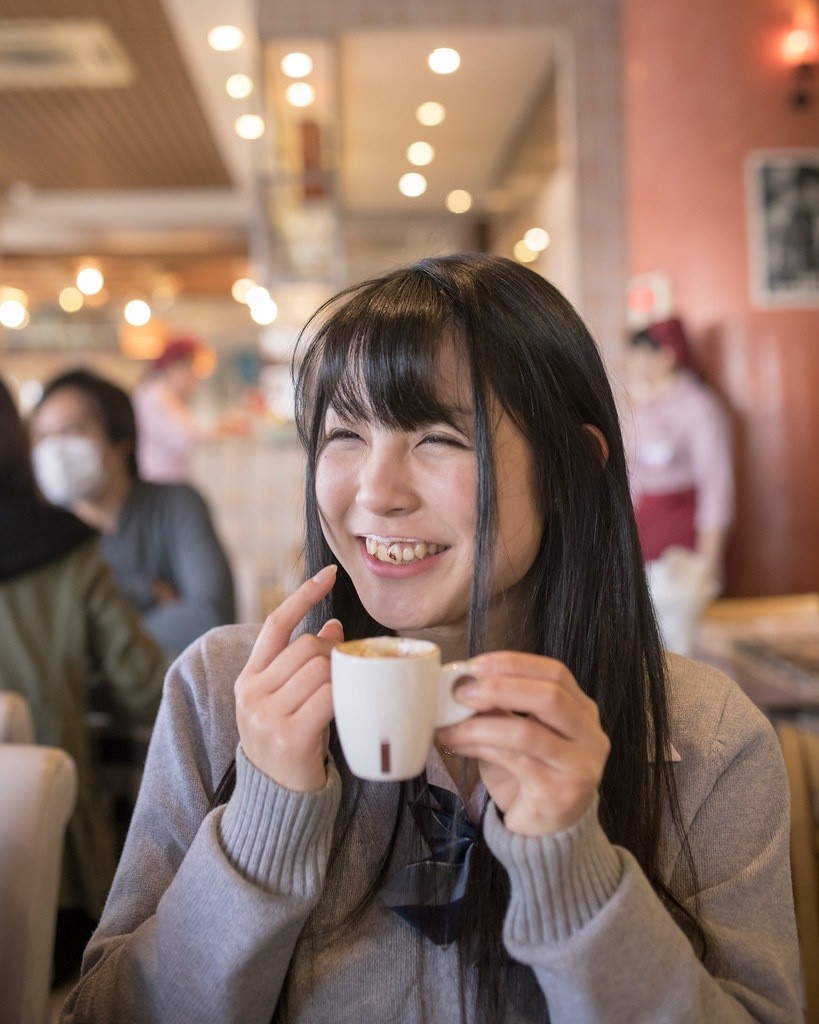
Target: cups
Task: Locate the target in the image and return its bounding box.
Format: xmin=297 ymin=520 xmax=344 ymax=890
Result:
xmin=329 ymin=636 xmax=479 ymax=783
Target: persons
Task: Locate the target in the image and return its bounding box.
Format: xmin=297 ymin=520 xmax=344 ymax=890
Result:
xmin=620 ymin=319 xmax=735 ymax=657
xmin=61 ymin=249 xmax=806 ymax=1024
xmin=0 ymin=333 xmax=240 ymax=1024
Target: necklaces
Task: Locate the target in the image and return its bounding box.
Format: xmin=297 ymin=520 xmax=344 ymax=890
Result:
xmin=442 ymin=741 xmax=457 ymax=758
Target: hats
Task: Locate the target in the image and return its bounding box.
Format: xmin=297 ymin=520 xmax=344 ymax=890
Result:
xmin=650 ymin=319 xmax=691 ymax=369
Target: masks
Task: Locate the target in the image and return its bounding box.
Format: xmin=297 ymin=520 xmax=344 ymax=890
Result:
xmin=30 ymin=434 xmax=103 ymax=506
